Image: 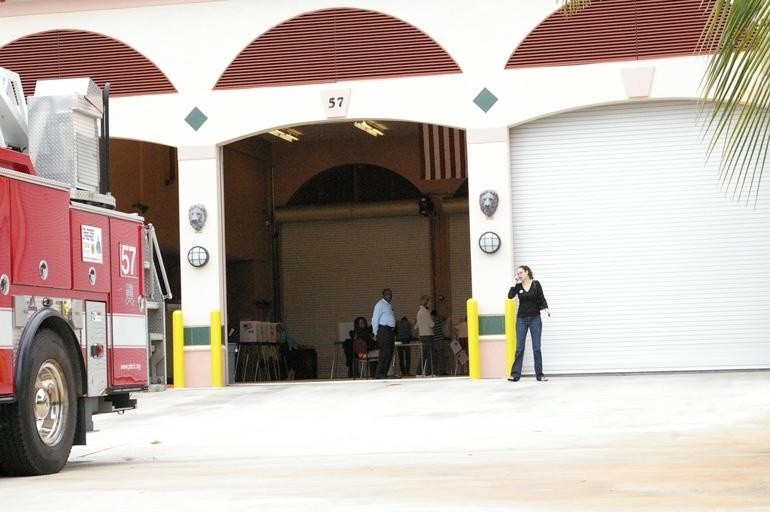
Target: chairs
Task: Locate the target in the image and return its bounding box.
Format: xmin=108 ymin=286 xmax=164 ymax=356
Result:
xmin=352 ymin=337 xmax=378 ymax=379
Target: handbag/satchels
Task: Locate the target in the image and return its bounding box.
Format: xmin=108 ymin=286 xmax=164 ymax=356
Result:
xmin=536 ymin=299 xmax=544 ymax=310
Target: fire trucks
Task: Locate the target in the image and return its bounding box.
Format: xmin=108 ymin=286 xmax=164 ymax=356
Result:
xmin=0 ymin=63 xmax=149 ymax=474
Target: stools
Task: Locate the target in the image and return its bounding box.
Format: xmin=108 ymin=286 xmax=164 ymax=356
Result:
xmin=329 ymin=342 xmax=348 ymax=380
xmin=234 ymin=342 xmax=280 ymax=382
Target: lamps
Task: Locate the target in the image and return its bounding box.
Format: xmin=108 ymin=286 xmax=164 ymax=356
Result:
xmin=479 ymin=232 xmax=500 ymax=254
xmin=188 ymin=246 xmax=209 ymax=267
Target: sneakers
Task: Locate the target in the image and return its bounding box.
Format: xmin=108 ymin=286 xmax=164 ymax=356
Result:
xmin=507 ymin=375 xmax=517 ymax=381
xmin=540 ymin=377 xmax=547 ymax=381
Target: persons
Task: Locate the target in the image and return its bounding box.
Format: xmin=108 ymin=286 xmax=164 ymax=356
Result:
xmin=507 ymin=265 xmax=551 ymax=382
xmin=342 ymin=288 xmax=468 ymax=379
xmin=276 ymin=324 xmax=302 ymax=380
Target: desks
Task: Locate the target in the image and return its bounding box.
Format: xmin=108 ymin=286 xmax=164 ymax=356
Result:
xmin=387 ymin=343 xmax=433 ymax=377
